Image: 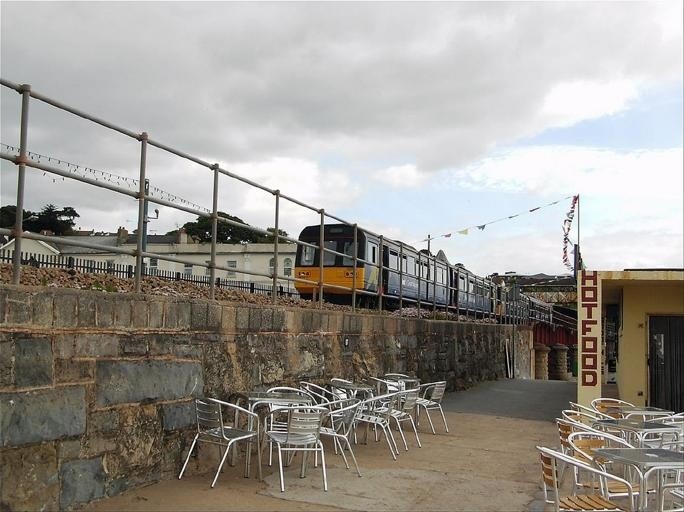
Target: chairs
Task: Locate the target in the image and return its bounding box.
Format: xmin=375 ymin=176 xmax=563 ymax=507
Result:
xmin=174 ymin=372 xmax=451 ymax=491
xmin=537 ymin=395 xmax=684 ymax=512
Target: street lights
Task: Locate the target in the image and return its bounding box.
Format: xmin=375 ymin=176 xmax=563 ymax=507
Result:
xmin=142 ymin=208 xmax=160 ymax=275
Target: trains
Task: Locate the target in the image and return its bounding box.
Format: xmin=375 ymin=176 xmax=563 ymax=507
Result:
xmin=292 ymin=223 xmax=553 ymax=325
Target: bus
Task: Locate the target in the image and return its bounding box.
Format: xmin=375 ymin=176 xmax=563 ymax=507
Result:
xmin=519 ymin=283 xmax=577 ymax=303
